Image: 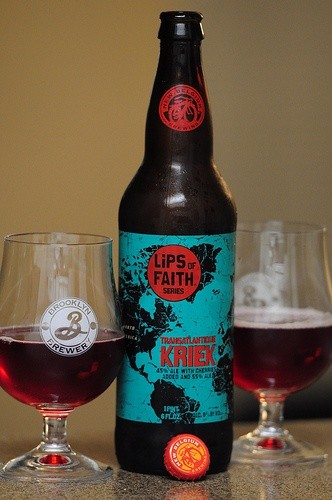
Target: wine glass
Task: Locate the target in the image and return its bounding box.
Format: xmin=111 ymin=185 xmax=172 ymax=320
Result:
xmin=0 ymin=232 xmax=123 ymax=483
xmin=231 ymin=222 xmax=332 ymax=465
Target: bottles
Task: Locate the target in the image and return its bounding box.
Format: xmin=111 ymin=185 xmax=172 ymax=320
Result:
xmin=118 ymin=11 xmax=230 ymax=478
